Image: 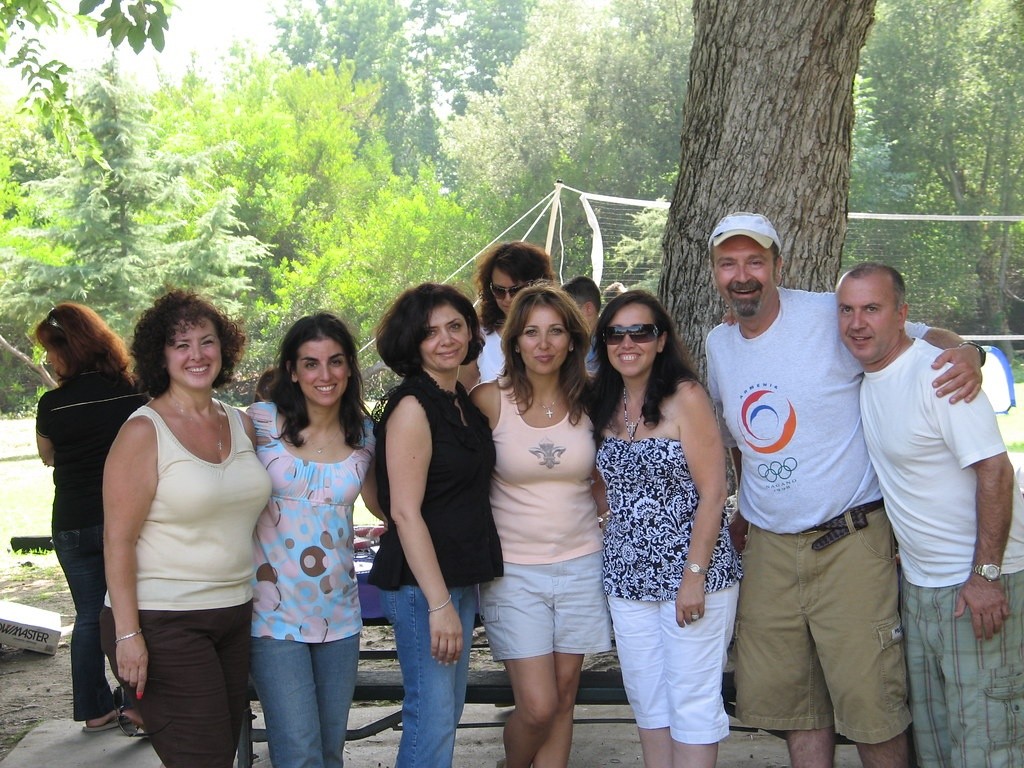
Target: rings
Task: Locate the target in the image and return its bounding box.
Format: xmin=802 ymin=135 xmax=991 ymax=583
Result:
xmin=692 ymin=615 xmax=700 ymax=620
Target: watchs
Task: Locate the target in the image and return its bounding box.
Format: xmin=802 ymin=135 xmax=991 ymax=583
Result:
xmin=973 ymin=564 xmax=1002 ymax=582
xmin=959 ymin=342 xmax=985 ymax=367
xmin=684 ymin=562 xmax=708 ymax=575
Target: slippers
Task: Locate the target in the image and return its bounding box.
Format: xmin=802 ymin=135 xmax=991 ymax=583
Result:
xmin=83 ymin=715 xmax=129 ymax=732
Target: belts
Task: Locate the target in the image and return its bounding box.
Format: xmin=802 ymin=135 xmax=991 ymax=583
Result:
xmin=800 ymin=499 xmax=884 ymax=550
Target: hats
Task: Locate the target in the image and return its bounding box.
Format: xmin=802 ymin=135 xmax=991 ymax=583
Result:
xmin=707 ymin=212 xmax=783 ymax=262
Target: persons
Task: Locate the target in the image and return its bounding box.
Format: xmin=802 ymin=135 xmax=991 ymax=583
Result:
xmin=706 ymin=211 xmax=983 ymax=768
xmin=102 ymin=290 xmax=271 ymax=768
xmin=247 ymin=316 xmax=388 ymax=768
xmin=837 ymin=263 xmax=1024 ymax=768
xmin=36 ymin=304 xmax=150 ymax=735
xmin=367 ymin=240 xmax=743 ymax=768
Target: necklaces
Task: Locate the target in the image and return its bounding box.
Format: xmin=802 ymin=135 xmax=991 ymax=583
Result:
xmin=308 ymin=428 xmax=341 ymax=453
xmin=534 ymin=391 xmax=561 ymax=418
xmin=623 ymin=382 xmax=644 ymax=442
xmin=170 ymin=389 xmax=223 ymax=448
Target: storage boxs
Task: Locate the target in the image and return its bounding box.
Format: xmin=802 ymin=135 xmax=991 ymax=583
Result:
xmin=0 ymin=597 xmax=62 ymax=658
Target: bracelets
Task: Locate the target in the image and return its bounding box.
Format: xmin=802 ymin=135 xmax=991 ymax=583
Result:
xmin=428 ymin=594 xmax=451 ymax=612
xmin=115 ymin=628 xmax=142 ymax=643
xmin=597 ymin=510 xmax=611 ymax=523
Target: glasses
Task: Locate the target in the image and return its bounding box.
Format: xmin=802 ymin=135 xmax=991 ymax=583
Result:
xmin=489 ymin=281 xmax=527 ymax=301
xmin=603 ymin=323 xmax=660 ymax=345
xmin=46 ymin=308 xmax=64 ymax=332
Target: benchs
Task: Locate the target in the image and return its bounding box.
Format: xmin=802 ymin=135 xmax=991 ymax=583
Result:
xmin=234 ymin=534 xmax=736 ymax=768
